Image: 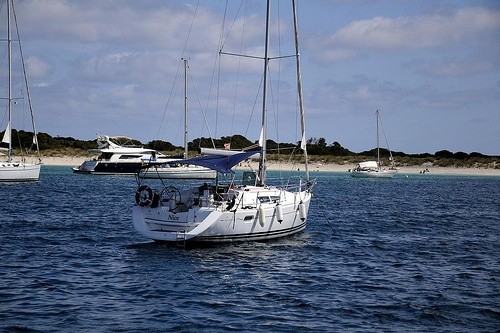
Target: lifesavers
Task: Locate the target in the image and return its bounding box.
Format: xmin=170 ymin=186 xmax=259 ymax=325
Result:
xmin=135 ymin=186 xmax=153 ymax=207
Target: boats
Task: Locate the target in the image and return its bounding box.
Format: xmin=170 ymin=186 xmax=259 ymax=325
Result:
xmin=71 ymin=133 xmax=172 ymax=178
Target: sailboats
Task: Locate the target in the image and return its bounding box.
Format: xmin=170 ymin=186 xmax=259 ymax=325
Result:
xmin=350 ymin=107 xmax=400 ymax=180
xmin=1 ymin=1 xmax=43 ymax=180
xmin=137 ymin=54 xmax=260 ymax=179
xmin=131 ymin=2 xmax=316 ymax=242
